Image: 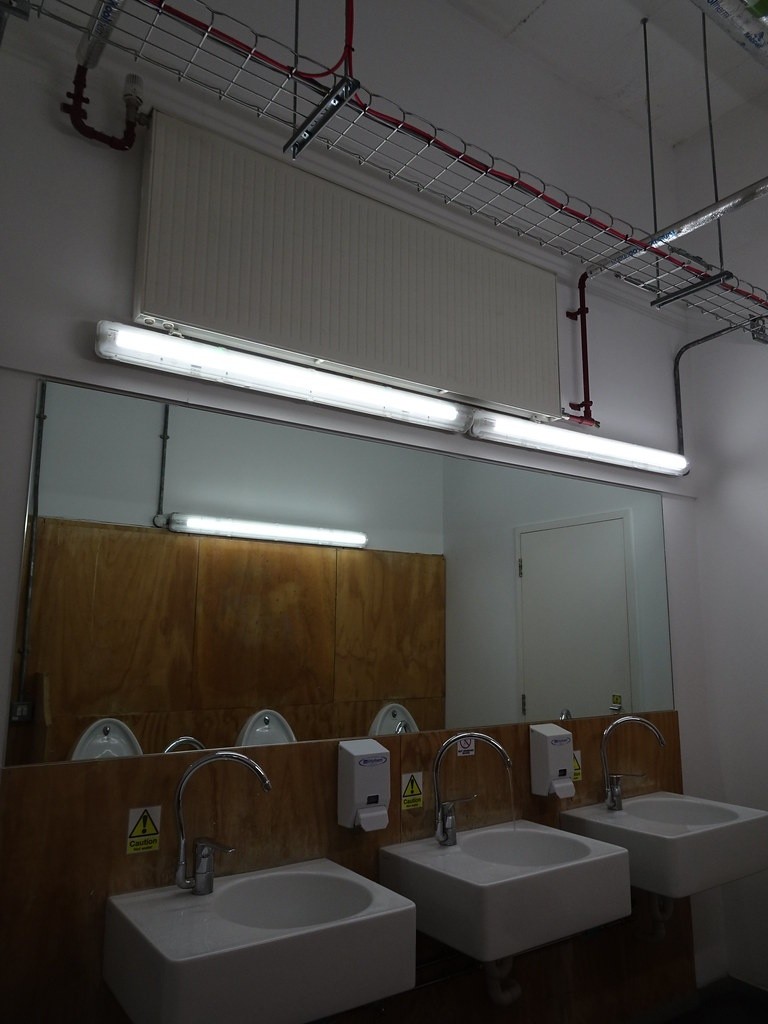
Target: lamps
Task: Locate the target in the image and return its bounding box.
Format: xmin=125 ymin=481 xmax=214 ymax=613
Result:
xmin=154 ymin=510 xmax=371 ymax=551
xmin=470 ymin=408 xmax=693 ymax=479
xmin=93 ymin=319 xmax=473 ymax=433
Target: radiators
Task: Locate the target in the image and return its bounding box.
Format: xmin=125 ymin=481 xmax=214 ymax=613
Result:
xmin=131 ymin=106 xmax=565 ymax=425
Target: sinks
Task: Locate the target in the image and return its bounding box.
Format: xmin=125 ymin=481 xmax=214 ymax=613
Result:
xmin=376 ymin=818 xmax=630 ymax=963
xmin=95 ymin=856 xmax=417 ymax=1024
xmin=559 ymin=790 xmax=768 ymax=899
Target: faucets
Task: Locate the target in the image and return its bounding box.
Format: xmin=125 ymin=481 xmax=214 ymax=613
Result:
xmin=173 ymin=750 xmax=274 ymax=895
xmin=433 ymin=732 xmax=513 ymax=846
xmin=560 ymin=709 xmax=572 ymax=720
xmin=394 ymin=719 xmax=412 ymax=733
xmin=161 ymin=735 xmax=208 ymax=754
xmin=599 ymin=715 xmax=666 ymax=811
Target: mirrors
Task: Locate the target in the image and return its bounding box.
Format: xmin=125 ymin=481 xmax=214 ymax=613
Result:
xmin=1 ymin=380 xmax=675 ymax=769
